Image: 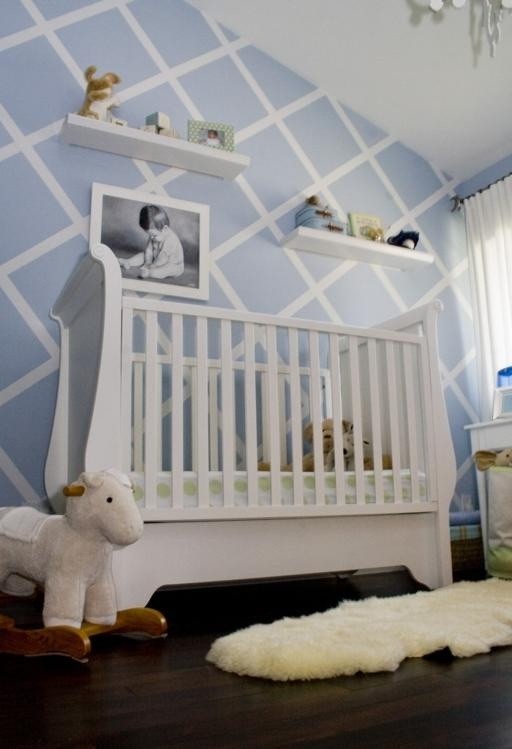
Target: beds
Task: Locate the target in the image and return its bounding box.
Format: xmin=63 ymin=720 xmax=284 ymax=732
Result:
xmin=42 ymin=243 xmax=457 ymax=639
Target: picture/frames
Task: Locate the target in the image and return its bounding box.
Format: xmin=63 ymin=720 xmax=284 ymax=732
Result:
xmin=89 ymin=180 xmax=210 ymax=302
xmin=187 ymin=118 xmax=236 ymax=152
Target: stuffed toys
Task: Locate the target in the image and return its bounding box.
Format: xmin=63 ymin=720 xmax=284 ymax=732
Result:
xmin=475 ymin=448 xmax=512 ymax=471
xmin=302 ymin=419 xmax=350 ymax=471
xmin=326 ymin=424 xmax=370 ymax=470
xmin=77 ymin=66 xmax=128 ymax=127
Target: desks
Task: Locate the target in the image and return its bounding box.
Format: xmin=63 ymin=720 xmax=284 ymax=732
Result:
xmin=462 ymin=418 xmax=511 ymax=558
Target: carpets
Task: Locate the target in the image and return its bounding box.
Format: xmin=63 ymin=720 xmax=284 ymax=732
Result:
xmin=206 ymin=575 xmax=512 ymax=682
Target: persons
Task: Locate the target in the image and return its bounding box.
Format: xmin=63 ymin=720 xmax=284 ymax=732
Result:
xmin=118 ymin=205 xmax=184 ymax=280
xmin=206 ymin=130 xmax=220 ymax=145
